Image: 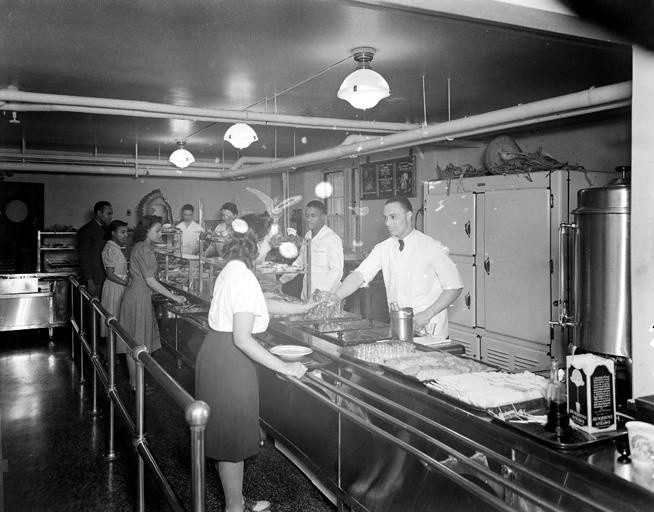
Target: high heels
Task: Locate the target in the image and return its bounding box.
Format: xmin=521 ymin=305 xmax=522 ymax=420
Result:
xmin=129 ymin=387 xmax=154 ymax=401
xmin=125 ymin=383 xmax=149 ymax=400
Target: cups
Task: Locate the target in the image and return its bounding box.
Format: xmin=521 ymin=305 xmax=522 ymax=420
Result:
xmin=301 ymin=288 xmax=345 ymax=322
xmin=625 ymin=420 xmax=654 ymax=466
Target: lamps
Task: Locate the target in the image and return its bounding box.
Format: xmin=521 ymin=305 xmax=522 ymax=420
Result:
xmin=168 ymin=139 xmax=197 ymax=171
xmin=335 ymin=46 xmax=395 ymax=111
xmin=222 ymin=122 xmax=258 ymax=150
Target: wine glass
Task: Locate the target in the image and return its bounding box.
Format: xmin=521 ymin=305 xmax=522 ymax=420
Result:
xmin=613 ymin=435 xmax=632 ymax=465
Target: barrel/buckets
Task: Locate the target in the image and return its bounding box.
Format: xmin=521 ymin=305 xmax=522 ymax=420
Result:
xmin=569 ymin=185 xmax=632 ymax=365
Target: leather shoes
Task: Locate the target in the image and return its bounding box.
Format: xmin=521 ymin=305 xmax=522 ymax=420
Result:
xmin=242 ymin=496 xmax=270 ymax=511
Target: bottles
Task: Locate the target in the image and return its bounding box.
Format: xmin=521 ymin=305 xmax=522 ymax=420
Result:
xmin=389 ymin=302 xmax=400 ymax=312
xmin=543 ymin=359 xmax=569 ymax=433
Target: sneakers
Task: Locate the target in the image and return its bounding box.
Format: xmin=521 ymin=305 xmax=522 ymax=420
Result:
xmin=243 ymin=505 xmax=271 ymax=512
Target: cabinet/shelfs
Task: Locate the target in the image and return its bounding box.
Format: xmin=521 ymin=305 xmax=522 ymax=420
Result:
xmin=195 ymin=227 xmax=312 ymax=307
xmin=38 ymin=230 xmax=138 ymax=277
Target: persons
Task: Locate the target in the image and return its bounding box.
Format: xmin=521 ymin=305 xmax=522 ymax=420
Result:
xmin=173 ymin=203 xmax=205 ymax=255
xmin=196 ymin=202 xmax=239 ymax=258
xmin=76 ymin=200 xmax=114 ymax=303
xmin=269 ymin=200 xmax=345 ymax=302
xmin=325 ymin=196 xmax=465 ymax=342
xmin=114 ymin=215 xmax=187 ymax=400
xmin=194 ymin=231 xmax=324 ymax=512
xmin=99 ymin=220 xmax=130 ymax=368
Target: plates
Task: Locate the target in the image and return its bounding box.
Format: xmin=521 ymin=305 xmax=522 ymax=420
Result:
xmin=484 ymin=135 xmax=517 ymax=174
xmin=269 ymin=344 xmax=314 ymax=361
xmin=160 ymin=228 xmax=177 ymax=234
xmin=153 ymin=243 xmax=169 ymax=247
